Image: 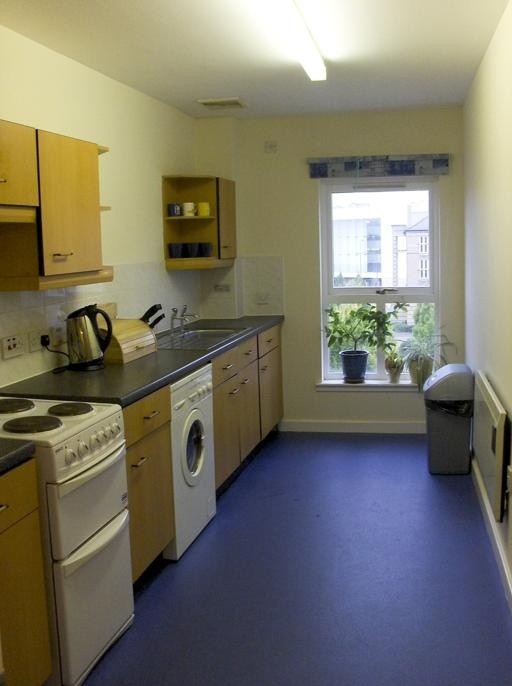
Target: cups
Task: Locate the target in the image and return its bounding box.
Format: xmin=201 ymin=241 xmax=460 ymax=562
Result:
xmin=168 ymin=242 xmax=213 ymax=258
xmin=166 ymin=202 xmax=211 ymax=217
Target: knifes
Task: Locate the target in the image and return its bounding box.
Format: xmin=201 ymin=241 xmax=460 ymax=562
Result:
xmin=140 ymin=304 xmax=165 ymax=329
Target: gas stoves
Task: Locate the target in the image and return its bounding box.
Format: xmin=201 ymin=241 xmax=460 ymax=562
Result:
xmin=0 ymin=398 xmax=121 ymax=444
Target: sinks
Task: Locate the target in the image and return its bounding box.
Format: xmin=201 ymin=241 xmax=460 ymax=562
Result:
xmin=157 ymin=326 xmax=253 ymax=350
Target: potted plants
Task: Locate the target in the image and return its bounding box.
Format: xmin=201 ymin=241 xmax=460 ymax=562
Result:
xmin=321 ymin=296 xmax=458 ymax=395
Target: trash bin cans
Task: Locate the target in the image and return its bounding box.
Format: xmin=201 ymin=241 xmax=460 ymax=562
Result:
xmin=422 ymin=363 xmax=475 ymax=476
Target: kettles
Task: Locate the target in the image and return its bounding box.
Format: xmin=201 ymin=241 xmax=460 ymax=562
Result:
xmin=63 ymin=304 xmax=112 ymax=372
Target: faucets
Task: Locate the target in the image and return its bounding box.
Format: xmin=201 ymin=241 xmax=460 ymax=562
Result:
xmin=170 ymin=307 xmax=193 ymax=337
xmin=180 ymin=305 xmax=200 ymax=334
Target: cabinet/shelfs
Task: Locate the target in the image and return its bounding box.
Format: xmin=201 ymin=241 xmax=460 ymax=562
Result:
xmin=122 ymin=382 xmax=178 ymax=586
xmin=0 ymin=118 xmax=41 ymax=226
xmin=210 ymin=334 xmax=262 ymax=493
xmin=0 ymin=117 xmax=115 ymax=293
xmin=2 ymin=458 xmax=55 ymax=686
xmin=256 ymin=321 xmax=284 ymax=441
xmin=160 ymin=173 xmax=238 ymax=269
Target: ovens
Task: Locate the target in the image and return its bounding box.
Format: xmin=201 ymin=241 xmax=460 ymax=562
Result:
xmin=46 ymin=412 xmax=134 ymax=686
